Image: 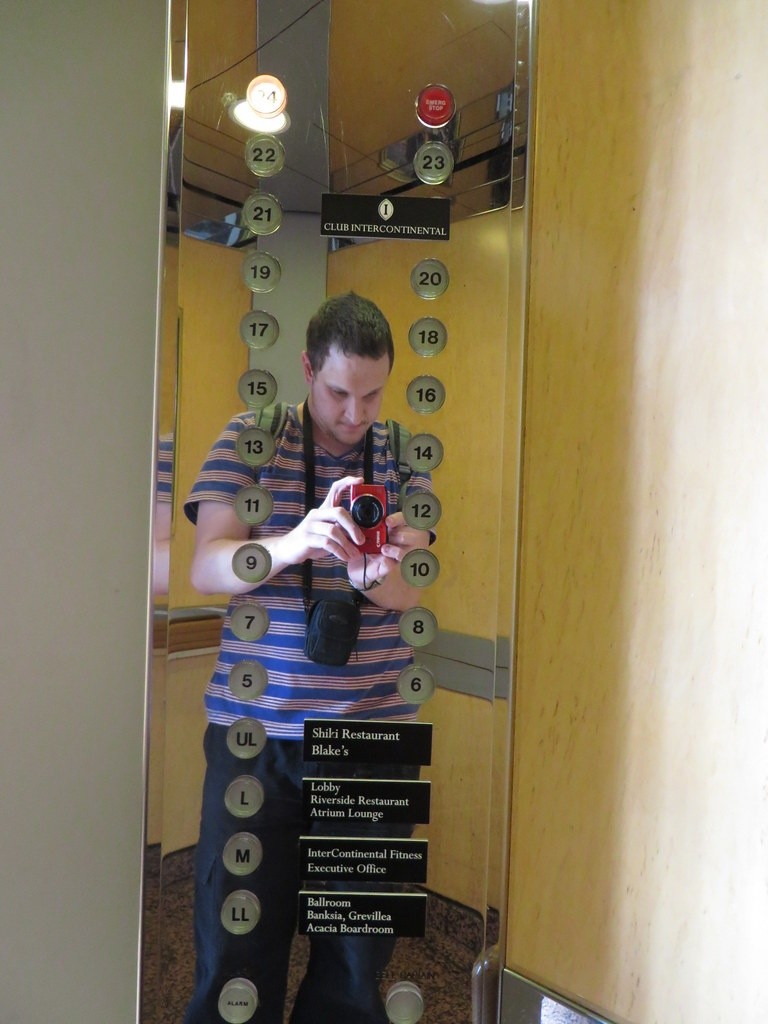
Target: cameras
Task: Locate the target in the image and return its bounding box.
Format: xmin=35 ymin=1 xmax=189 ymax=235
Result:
xmin=350 ymin=484 xmax=388 ymax=553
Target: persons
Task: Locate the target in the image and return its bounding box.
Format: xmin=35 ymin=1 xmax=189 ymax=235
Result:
xmin=183 ymin=291 xmax=437 ymax=1024
xmin=153 ymin=433 xmax=174 ymax=593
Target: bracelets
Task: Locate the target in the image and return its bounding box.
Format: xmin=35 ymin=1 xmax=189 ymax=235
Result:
xmin=349 ymin=577 xmax=384 ymax=591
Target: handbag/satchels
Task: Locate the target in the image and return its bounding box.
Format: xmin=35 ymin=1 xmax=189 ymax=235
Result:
xmin=304 ymin=597 xmax=362 ymax=665
